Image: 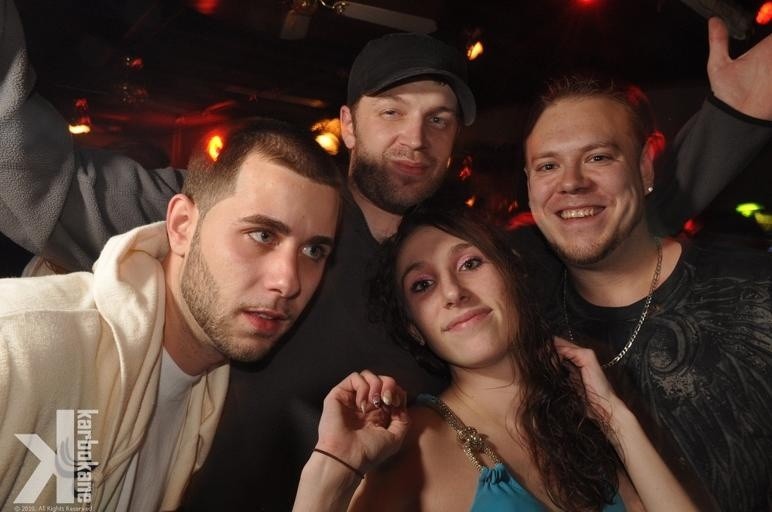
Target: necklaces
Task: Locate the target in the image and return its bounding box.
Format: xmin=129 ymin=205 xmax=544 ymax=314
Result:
xmin=563 ymin=232 xmax=663 ymax=370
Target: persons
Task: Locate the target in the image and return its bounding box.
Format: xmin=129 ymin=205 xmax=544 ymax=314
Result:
xmin=1 ymin=117 xmax=343 ymax=511
xmin=0 ymin=0 xmax=772 ymax=510
xmin=504 ymin=66 xmax=772 ymax=511
xmin=291 ymin=196 xmax=703 ymax=511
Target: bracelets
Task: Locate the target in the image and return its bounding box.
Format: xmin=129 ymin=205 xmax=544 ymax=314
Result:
xmin=312 ymin=446 xmax=366 ymax=480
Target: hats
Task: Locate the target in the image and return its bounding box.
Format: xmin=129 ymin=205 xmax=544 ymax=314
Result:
xmin=347 ymin=33 xmax=477 ymax=127
